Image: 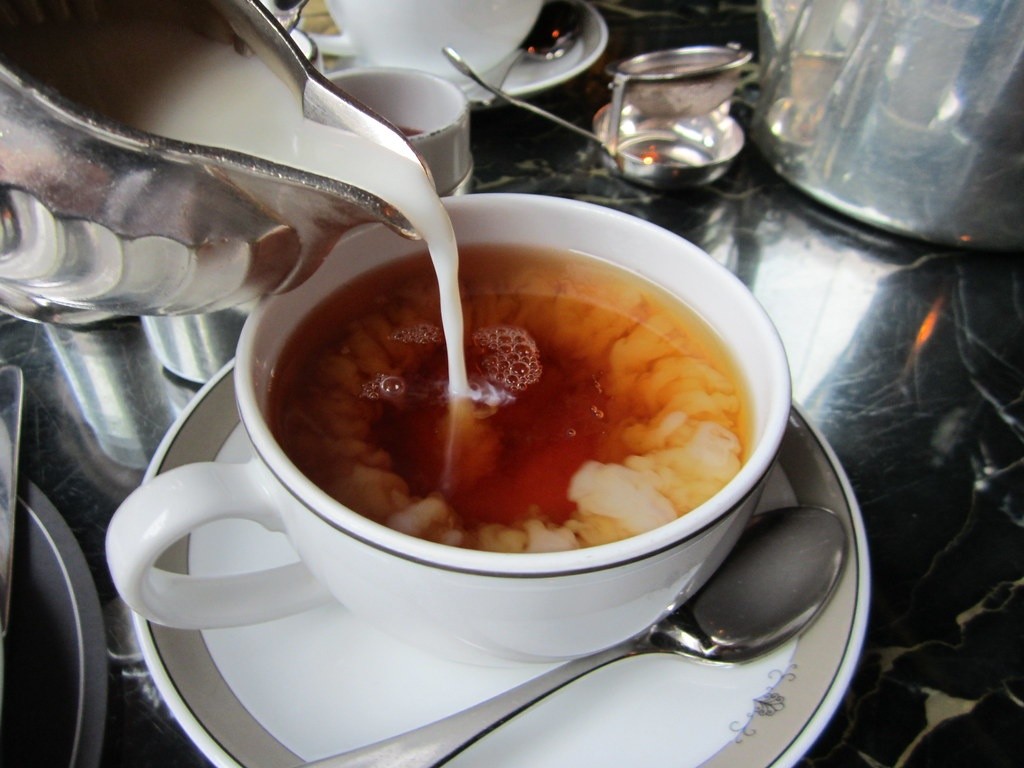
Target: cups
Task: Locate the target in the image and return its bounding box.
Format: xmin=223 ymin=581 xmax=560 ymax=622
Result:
xmin=301 ymin=1 xmax=545 ymax=77
xmin=105 ymin=189 xmax=794 ymax=664
xmin=323 ymin=67 xmax=474 ymax=198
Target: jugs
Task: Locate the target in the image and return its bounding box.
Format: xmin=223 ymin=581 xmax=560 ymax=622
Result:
xmin=0 ymin=0 xmax=440 ymax=328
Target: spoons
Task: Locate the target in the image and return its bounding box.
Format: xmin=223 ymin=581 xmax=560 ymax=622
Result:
xmin=466 ymin=0 xmax=584 ymax=120
xmin=290 ymin=505 xmax=850 ymax=768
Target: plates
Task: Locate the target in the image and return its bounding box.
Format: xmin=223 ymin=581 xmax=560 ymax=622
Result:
xmin=128 ymin=354 xmax=873 ymax=768
xmin=459 ymin=0 xmax=608 ymax=108
